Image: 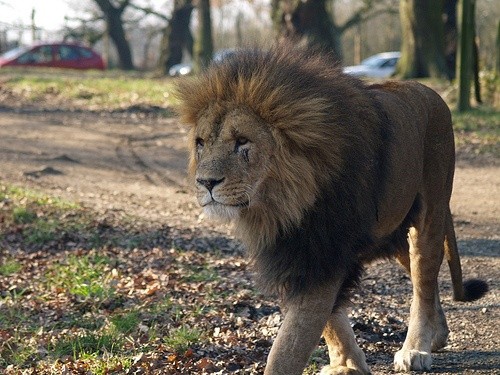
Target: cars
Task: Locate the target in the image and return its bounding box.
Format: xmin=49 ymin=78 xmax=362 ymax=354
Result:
xmin=342 ymin=50 xmax=403 ymax=80
xmin=0 ymin=40 xmax=106 ymax=70
xmin=169 ymin=48 xmax=249 ymax=78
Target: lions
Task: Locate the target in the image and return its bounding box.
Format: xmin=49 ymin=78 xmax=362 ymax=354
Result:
xmin=172 ymin=38 xmax=489 ymax=375
